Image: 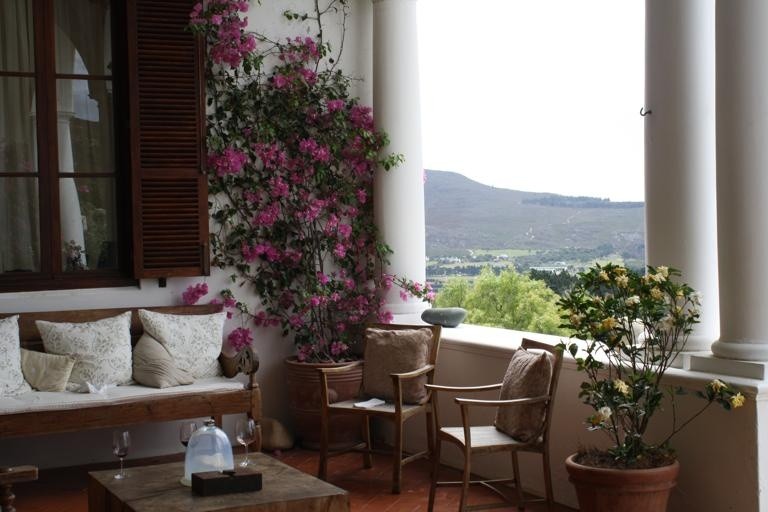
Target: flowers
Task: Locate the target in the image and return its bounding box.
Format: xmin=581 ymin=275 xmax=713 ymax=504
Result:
xmin=551 ymin=261 xmax=746 ymax=460
xmin=180 ymin=0 xmax=442 ymax=362
xmin=61 ymin=241 xmax=87 ymax=273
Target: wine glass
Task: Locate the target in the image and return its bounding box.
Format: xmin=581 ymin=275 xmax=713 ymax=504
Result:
xmin=236 ymin=415 xmax=257 ymax=471
xmin=179 ymin=421 xmax=198 ymax=452
xmin=110 ymin=428 xmax=131 ymax=481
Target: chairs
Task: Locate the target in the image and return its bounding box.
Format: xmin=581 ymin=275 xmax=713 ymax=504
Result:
xmin=314 ymin=320 xmax=445 ymax=496
xmin=423 ymin=336 xmax=566 ymax=510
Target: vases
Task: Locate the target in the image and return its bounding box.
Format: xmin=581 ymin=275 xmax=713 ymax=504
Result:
xmin=563 ymin=449 xmax=681 ymax=512
xmin=282 ymin=351 xmax=372 ymax=450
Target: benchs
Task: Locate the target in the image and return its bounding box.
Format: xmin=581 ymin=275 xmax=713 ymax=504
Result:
xmin=0 ymin=301 xmax=266 ymax=455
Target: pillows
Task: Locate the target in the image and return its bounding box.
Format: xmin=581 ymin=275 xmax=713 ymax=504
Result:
xmin=34 ymin=310 xmax=135 ymax=394
xmin=136 ymin=307 xmax=229 ymax=379
xmin=361 ymin=326 xmax=430 ymax=405
xmin=21 ymin=348 xmax=76 ymax=395
xmin=131 ymin=330 xmax=194 ymax=388
xmin=0 ymin=312 xmax=34 ymax=398
xmin=494 ymin=343 xmax=553 ymax=442
